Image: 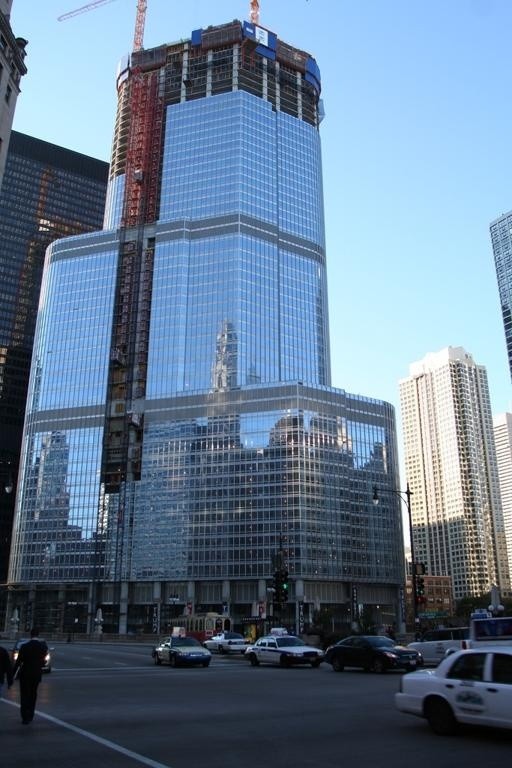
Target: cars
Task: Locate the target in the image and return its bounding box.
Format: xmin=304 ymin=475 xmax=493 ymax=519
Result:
xmin=153 ymin=635 xmax=211 ymax=668
xmin=12 ymin=638 xmax=54 ymax=674
xmin=245 ymin=628 xmax=325 ymax=668
xmin=407 ymin=627 xmax=472 ymax=664
xmin=325 ymin=636 xmax=424 ymax=672
xmin=203 ymin=632 xmax=245 ymax=655
xmin=394 ymin=648 xmax=512 ymax=733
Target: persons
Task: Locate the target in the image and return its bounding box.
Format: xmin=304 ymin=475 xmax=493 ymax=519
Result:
xmin=0 ymin=645 xmax=15 ymax=690
xmin=12 ymin=627 xmax=48 ymax=726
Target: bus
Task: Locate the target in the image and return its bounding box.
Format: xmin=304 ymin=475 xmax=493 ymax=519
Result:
xmin=167 ymin=611 xmax=231 ymax=642
xmin=470 ymin=616 xmax=512 ymax=649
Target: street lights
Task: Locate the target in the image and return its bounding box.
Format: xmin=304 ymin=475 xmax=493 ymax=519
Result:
xmin=67 ymin=598 xmax=78 ymax=641
xmin=487 ymin=604 xmax=506 ymax=617
xmin=371 ymin=482 xmax=421 ymax=640
xmin=276 ymin=532 xmax=288 ymax=626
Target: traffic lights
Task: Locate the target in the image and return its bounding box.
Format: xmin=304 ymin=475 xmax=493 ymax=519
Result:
xmin=271 ymin=574 xmax=280 ymax=604
xmin=416 ymin=578 xmax=426 ymax=599
xmin=282 ymin=574 xmax=289 ymax=603
